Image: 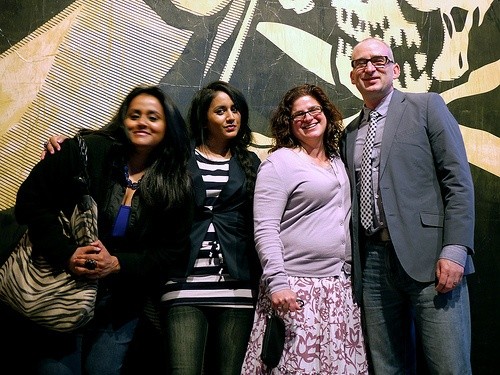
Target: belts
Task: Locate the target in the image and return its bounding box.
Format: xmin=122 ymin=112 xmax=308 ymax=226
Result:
xmin=362 ymin=227 xmax=391 ymax=242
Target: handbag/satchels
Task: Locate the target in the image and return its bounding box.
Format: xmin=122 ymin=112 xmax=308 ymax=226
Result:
xmin=0 ymin=134 xmax=99 ymax=331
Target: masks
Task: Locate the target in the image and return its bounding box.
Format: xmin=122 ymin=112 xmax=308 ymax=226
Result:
xmin=260 ymin=308 xmax=285 ymax=374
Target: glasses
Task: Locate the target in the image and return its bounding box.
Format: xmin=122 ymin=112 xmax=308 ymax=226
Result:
xmin=351 ymin=55 xmax=394 ymax=69
xmin=290 ymin=106 xmax=323 ymax=120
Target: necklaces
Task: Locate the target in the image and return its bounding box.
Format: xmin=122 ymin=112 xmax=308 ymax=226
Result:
xmin=124 ymin=165 xmax=145 ymax=189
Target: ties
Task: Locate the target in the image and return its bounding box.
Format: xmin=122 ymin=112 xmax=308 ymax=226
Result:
xmin=359 ymin=110 xmax=379 ymax=230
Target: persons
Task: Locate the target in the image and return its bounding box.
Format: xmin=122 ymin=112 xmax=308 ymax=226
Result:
xmin=241 ymin=84 xmax=369 ymax=375
xmin=338 ymin=38 xmax=475 ymax=375
xmin=41 ymin=81 xmax=261 ymax=375
xmin=14 ymin=87 xmax=194 ymax=375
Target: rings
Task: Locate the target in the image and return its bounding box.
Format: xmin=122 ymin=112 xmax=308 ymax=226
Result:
xmin=44 ymin=141 xmax=48 ymax=148
xmin=84 ymin=259 xmax=97 ymax=268
xmin=453 ymin=282 xmax=457 ymax=285
xmin=278 ymin=305 xmax=283 ymax=308
